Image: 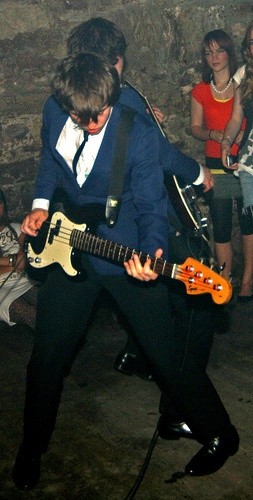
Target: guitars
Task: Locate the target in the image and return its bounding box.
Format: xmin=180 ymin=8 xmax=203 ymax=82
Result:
xmin=23 ymin=188 xmax=234 ymax=304
xmin=124 ymin=79 xmax=211 ymax=245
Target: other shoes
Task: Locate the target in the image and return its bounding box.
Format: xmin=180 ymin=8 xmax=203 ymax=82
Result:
xmin=237 ymin=294 xmax=251 ymax=302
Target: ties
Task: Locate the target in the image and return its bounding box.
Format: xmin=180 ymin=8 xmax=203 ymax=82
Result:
xmin=73 ymin=131 xmax=89 ymax=178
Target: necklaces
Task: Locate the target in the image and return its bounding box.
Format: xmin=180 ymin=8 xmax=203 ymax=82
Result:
xmin=211 ymin=77 xmax=232 ymax=94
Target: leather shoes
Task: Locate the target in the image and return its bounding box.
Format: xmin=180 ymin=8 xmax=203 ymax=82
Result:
xmin=12 ymin=441 xmax=41 ymax=491
xmin=158 ymin=417 xmax=240 ymax=476
xmin=114 ymin=350 xmax=153 ymax=381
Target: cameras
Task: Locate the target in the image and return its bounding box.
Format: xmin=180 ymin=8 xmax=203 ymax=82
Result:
xmin=227 ymin=155 xmax=235 ymax=166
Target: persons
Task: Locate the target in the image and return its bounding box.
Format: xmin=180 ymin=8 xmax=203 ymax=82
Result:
xmin=13 ymin=52 xmax=240 ymax=491
xmin=190 ymin=29 xmax=253 ymax=301
xmin=221 ymin=22 xmax=253 ymax=216
xmin=0 ymin=190 xmax=83 ymax=353
xmin=67 ymin=17 xmax=215 ymax=440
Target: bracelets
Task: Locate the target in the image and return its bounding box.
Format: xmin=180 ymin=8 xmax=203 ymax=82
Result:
xmin=209 ymin=129 xmax=213 ymax=140
xmin=222 ymin=138 xmax=233 ymax=145
xmin=9 ymin=255 xmax=14 ymax=267
